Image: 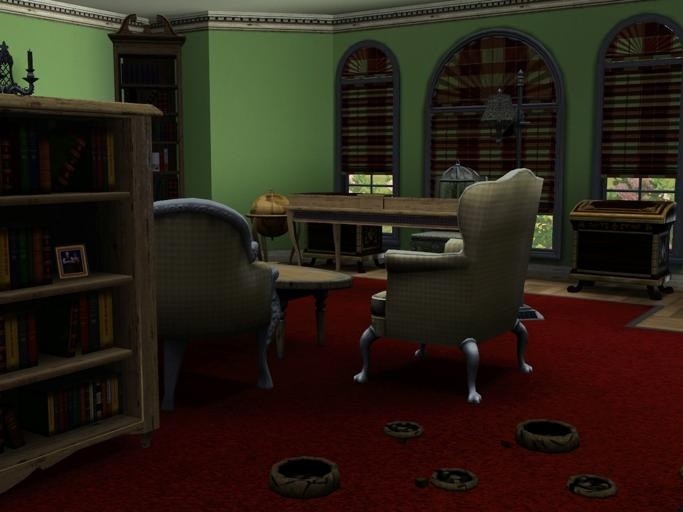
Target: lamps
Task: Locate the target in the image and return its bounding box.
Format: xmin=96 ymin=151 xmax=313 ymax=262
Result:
xmin=480 ymin=88 xmax=523 ymax=144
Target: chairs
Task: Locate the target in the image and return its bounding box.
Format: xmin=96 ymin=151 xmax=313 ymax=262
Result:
xmin=353 ymin=167 xmax=546 ymax=404
xmin=151 ymin=197 xmax=282 ymax=414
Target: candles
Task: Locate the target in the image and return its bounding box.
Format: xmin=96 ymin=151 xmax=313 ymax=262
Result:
xmin=26 ymin=48 xmax=34 ymax=69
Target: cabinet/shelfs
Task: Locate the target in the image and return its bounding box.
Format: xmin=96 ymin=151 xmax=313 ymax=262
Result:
xmin=107 ymin=14 xmax=186 ymax=202
xmin=0 ymin=91 xmax=164 ymax=495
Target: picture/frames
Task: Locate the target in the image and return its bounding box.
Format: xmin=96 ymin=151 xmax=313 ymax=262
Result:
xmin=55 ymin=243 xmax=89 ymax=280
